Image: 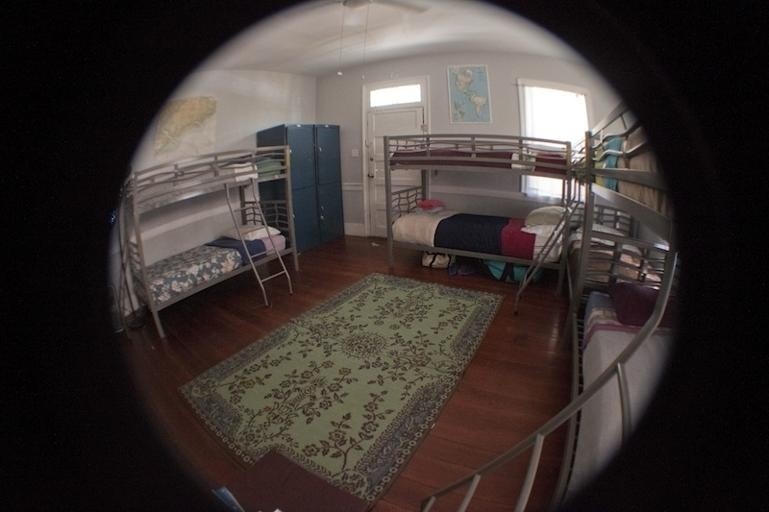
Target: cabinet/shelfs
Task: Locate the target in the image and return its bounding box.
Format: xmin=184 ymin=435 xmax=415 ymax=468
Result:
xmin=256 ymin=124 xmax=345 ymax=252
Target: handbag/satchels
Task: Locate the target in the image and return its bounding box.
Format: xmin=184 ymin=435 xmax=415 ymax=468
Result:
xmin=488 ymin=261 xmax=543 ymax=284
xmin=422 ymin=251 xmax=450 ymax=269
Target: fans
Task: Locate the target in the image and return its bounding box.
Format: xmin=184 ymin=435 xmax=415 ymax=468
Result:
xmin=291 ymin=0 xmax=428 ymax=19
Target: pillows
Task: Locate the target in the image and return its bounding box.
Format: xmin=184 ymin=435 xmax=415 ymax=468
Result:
xmin=221 ymin=223 xmax=282 ymax=243
xmin=523 ymin=205 xmax=568 ymax=225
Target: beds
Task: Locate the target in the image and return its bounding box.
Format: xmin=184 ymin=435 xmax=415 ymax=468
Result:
xmin=111 ymin=143 xmax=300 ymax=338
xmin=382 ymin=132 xmax=572 ymax=295
